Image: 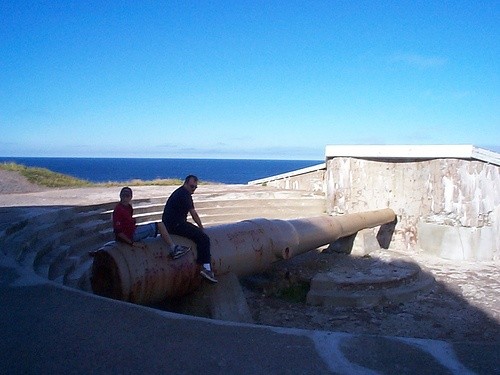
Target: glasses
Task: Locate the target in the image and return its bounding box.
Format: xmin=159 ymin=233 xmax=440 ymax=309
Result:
xmin=187 ymin=183 xmax=197 ymax=188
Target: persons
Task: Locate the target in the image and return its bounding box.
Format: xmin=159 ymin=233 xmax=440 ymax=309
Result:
xmin=162 ymin=174 xmax=219 ymax=282
xmin=113 ymin=186 xmax=192 ymax=260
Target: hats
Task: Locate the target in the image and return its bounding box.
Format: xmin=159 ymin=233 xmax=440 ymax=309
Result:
xmin=120 ymin=187 xmax=132 ymax=195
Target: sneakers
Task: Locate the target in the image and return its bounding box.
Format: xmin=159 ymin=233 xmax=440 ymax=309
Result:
xmin=169 ymin=245 xmax=192 ymax=260
xmin=200 ymin=267 xmax=218 ymax=282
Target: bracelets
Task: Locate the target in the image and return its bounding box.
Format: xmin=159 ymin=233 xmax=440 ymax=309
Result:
xmin=130 ymin=241 xmax=135 ymax=247
